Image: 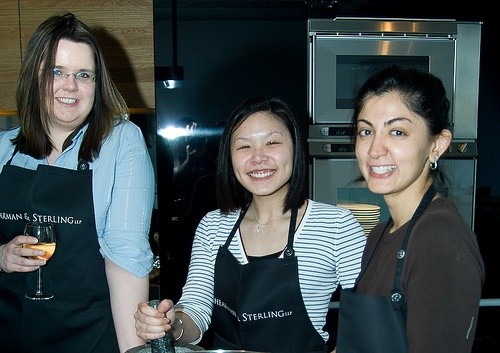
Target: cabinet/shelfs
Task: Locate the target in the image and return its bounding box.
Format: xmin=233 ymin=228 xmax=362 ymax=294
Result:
xmin=0 ymin=0 xmax=155 ymax=115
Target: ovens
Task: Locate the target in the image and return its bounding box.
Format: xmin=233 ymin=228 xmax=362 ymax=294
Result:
xmin=307 ymin=19 xmax=483 ymax=231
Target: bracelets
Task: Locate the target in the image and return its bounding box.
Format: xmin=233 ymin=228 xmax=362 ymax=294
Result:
xmin=175 ymin=315 xmax=184 ymax=341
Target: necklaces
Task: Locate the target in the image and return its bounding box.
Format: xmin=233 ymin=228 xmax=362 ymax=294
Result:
xmin=250 ymin=206 xmax=282 ymax=233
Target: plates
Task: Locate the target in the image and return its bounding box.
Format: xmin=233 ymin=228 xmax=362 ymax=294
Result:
xmin=337 ymin=204 xmax=380 ymax=238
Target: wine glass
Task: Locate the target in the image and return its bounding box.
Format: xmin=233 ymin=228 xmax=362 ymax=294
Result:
xmin=24 ymin=223 xmax=56 ymax=300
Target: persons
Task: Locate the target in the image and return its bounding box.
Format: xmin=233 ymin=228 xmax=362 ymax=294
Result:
xmin=134 ymin=98 xmax=367 ymax=353
xmin=337 ymin=68 xmax=485 ymax=353
xmin=0 ymin=13 xmax=155 ymax=353
